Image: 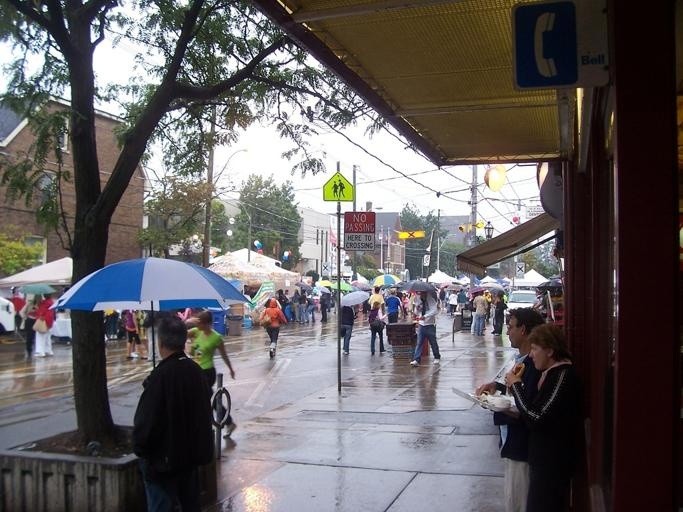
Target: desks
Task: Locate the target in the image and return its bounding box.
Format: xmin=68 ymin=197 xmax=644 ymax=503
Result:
xmin=51 ymin=311 xmax=73 ymax=343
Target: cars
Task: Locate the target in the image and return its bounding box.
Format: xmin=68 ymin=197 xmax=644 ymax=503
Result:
xmin=504 ymin=289 xmax=539 ymax=324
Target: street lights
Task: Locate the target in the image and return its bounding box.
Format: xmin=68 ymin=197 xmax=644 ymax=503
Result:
xmin=485 ymin=221 xmax=495 ymax=242
xmin=350 ymin=207 xmax=384 ymax=281
xmin=202 ymin=147 xmax=248 ymax=271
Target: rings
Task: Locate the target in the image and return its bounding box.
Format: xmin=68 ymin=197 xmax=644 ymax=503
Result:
xmin=479 ymin=386 xmax=481 ymax=391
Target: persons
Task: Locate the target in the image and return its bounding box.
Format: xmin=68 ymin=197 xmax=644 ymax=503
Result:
xmin=18 ymin=293 xmax=42 ymax=357
xmin=126 ymin=309 xmax=148 ymax=360
xmin=28 ymin=293 xmax=57 ymax=357
xmin=182 ymin=310 xmax=236 ymax=439
xmin=504 ymin=320 xmax=592 ymax=512
xmin=260 ymin=286 xmax=508 ymax=368
xmin=476 ymin=307 xmax=546 ymax=512
xmin=132 ymin=314 xmax=215 ymax=512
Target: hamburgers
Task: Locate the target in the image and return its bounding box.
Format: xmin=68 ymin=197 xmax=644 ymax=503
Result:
xmin=512 ymin=361 xmax=526 ymax=377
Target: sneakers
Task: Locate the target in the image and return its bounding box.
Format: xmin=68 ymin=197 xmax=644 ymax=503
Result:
xmin=269 ymin=349 xmax=274 ymax=358
xmin=433 ymin=358 xmax=441 ymax=364
xmin=409 ymin=360 xmax=420 ymax=366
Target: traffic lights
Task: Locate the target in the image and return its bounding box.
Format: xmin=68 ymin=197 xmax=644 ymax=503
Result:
xmin=457 ymin=224 xmax=466 ymax=233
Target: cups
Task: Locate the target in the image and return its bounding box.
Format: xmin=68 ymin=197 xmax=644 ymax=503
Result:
xmin=482 ymin=393 xmax=516 ymax=409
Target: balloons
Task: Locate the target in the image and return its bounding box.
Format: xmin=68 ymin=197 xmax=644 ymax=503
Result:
xmin=254 ymin=240 xmax=259 ymax=246
xmin=257 ymin=243 xmax=262 ymax=249
xmin=229 ymin=218 xmax=235 ymax=224
xmin=284 ymin=251 xmax=289 ymax=256
xmin=283 ymin=255 xmax=289 ymax=260
xmin=226 ymin=229 xmax=232 ymax=236
xmin=257 ymin=249 xmax=263 ymax=254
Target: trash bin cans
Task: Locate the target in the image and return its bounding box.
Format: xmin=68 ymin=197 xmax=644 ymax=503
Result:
xmin=451 ymin=311 xmax=462 ymax=331
xmin=206 ymin=306 xmax=227 ymax=335
xmin=226 ymin=315 xmax=243 ymax=336
xmin=284 ymin=303 xmax=293 ymax=321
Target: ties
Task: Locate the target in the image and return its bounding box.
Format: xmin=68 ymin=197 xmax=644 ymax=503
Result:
xmin=420 ymin=298 xmax=425 ymax=319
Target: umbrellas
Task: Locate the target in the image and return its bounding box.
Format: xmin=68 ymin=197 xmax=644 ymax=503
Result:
xmin=16 ymin=284 xmax=56 ymax=295
xmin=48 ymin=255 xmax=253 ymax=370
xmin=295 ymin=274 xmax=509 ymax=308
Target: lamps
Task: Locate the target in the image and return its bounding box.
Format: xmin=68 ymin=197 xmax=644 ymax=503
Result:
xmin=536 ymin=162 xmax=550 ymax=189
xmin=483 ymin=167 xmax=505 ymax=192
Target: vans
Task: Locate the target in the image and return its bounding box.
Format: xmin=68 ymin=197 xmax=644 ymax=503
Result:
xmin=0 ymin=296 xmax=15 ymax=335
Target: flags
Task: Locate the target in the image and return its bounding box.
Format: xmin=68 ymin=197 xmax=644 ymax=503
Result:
xmin=329 ymin=229 xmax=336 ymax=244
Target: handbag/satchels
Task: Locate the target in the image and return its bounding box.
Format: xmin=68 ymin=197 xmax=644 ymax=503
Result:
xmin=370 ymin=320 xmax=386 ymax=331
xmin=31 ymin=317 xmax=47 ymax=332
xmin=259 ymin=311 xmax=271 ymax=327
